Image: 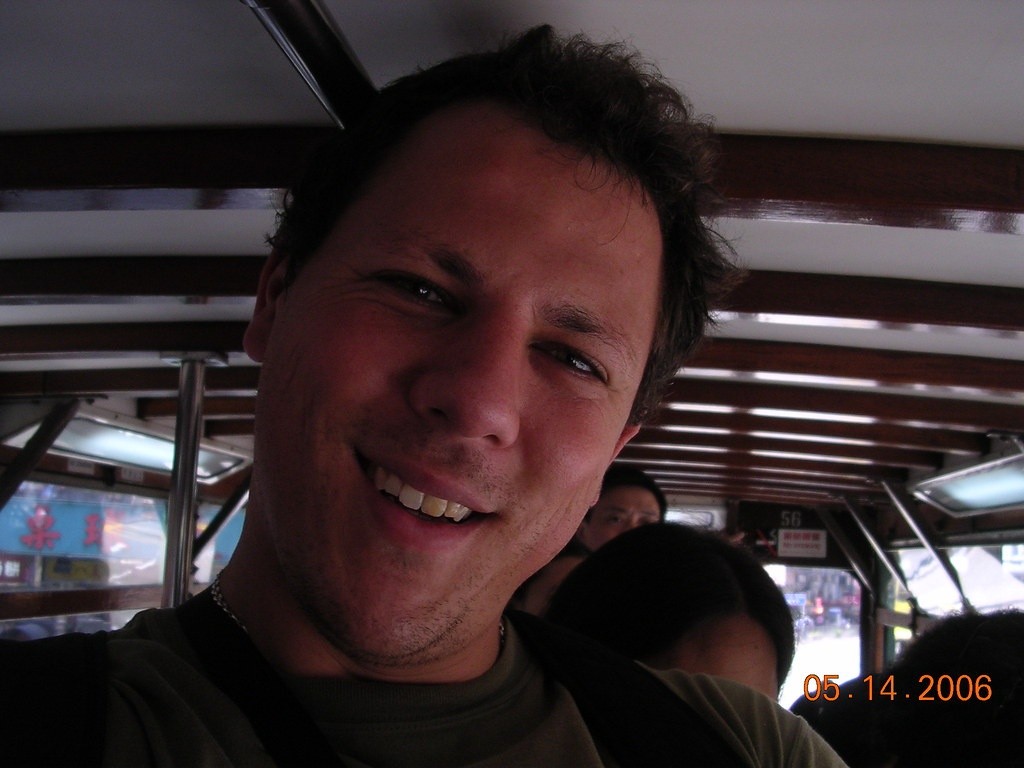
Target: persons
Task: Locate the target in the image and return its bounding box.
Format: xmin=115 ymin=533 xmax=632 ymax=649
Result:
xmin=789 ymin=610 xmax=1024 ymax=768
xmin=512 ymin=462 xmax=667 ymax=612
xmin=2 ymin=23 xmax=852 ymax=767
xmin=541 ymin=521 xmax=794 ymax=700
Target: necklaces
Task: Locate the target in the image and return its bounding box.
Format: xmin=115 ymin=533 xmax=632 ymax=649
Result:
xmin=212 ymin=569 xmax=247 ymax=632
